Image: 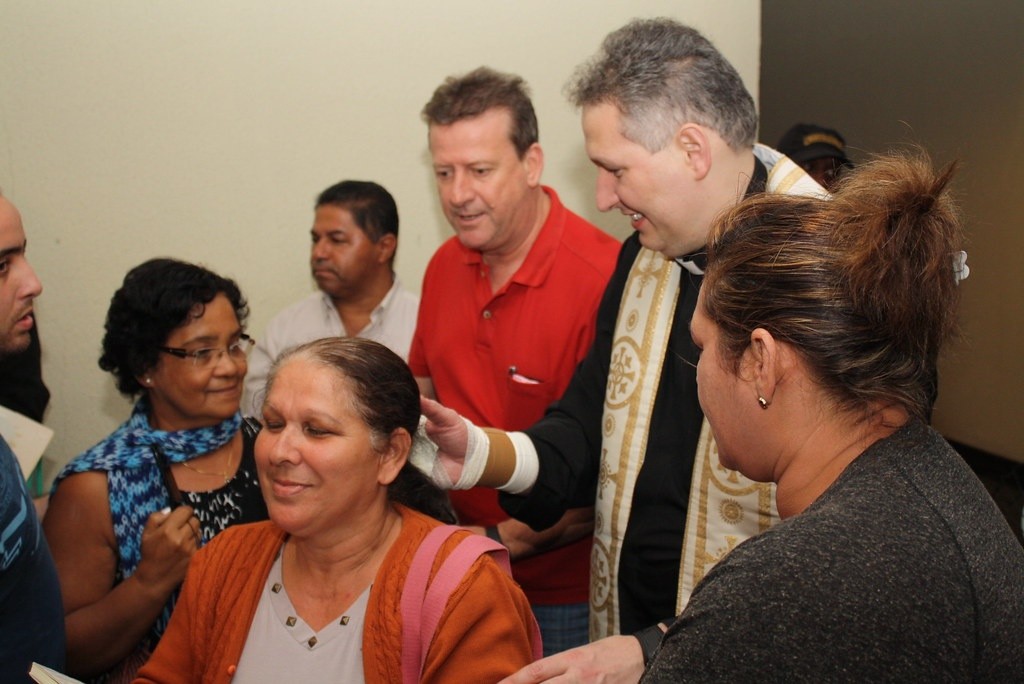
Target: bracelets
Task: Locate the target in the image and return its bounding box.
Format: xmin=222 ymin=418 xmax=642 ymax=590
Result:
xmin=485 ymin=523 xmax=504 ymax=546
xmin=638 ymin=627 xmax=667 ymax=669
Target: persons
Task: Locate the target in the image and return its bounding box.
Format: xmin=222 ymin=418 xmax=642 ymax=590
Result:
xmin=240 ymin=181 xmax=422 ymax=418
xmin=778 ymin=123 xmax=856 ymax=191
xmin=0 ymin=193 xmax=44 ymax=684
xmin=407 ymin=67 xmax=622 ymax=659
xmin=41 ymin=257 xmax=269 ymax=684
xmin=417 ymin=17 xmax=834 ymax=684
xmin=636 ymin=141 xmax=1024 ymax=684
xmin=132 ymin=337 xmax=543 ymax=684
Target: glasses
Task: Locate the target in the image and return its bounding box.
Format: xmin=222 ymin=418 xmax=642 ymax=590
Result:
xmin=157 ymin=333 xmax=255 ymax=367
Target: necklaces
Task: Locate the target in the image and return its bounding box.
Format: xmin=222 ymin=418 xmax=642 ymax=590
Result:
xmin=182 ymin=439 xmax=237 ymax=486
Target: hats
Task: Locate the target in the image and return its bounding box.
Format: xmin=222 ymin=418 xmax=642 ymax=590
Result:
xmin=777 ymin=125 xmax=855 ymax=171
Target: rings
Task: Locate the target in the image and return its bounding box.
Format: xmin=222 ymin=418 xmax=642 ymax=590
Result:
xmin=187 ymin=522 xmax=196 ymax=535
xmin=194 ymin=535 xmax=200 ymax=546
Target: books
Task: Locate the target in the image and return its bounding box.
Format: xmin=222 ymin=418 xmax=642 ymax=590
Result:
xmin=28 ymin=662 xmax=85 ymax=684
xmin=0 ymin=406 xmax=54 ymax=485
